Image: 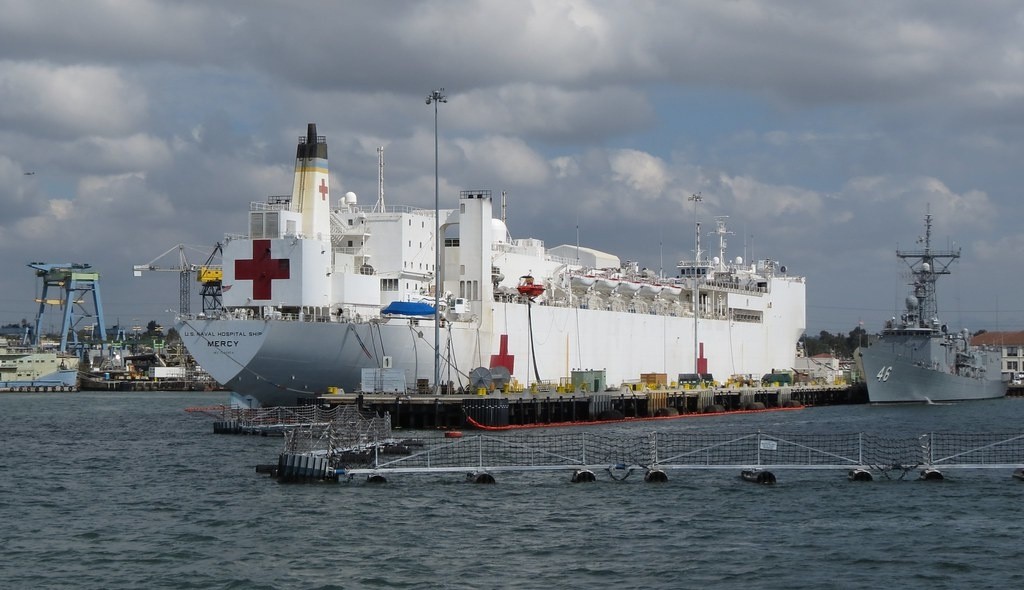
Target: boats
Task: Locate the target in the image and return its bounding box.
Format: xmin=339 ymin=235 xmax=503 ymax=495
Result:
xmin=856 ymin=200 xmax=1010 ymax=404
xmin=167 ymin=121 xmax=807 ymax=408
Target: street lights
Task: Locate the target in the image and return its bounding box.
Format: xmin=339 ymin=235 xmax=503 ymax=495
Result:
xmin=419 ymin=83 xmax=448 ymax=403
